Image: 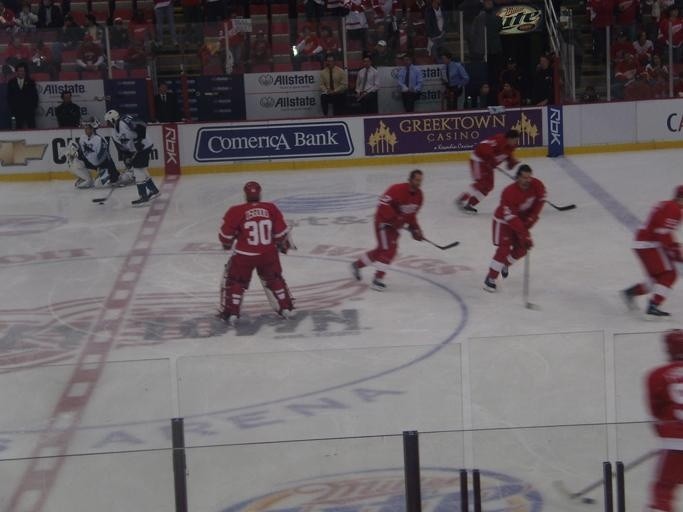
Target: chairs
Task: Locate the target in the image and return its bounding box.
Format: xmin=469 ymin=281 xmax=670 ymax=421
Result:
xmin=0 ymin=1 xmax=434 ymax=82
xmin=583 ymin=3 xmax=636 ymax=32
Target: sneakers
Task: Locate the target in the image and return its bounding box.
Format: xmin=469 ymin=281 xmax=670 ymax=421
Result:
xmin=453 ymin=196 xmax=466 ymax=208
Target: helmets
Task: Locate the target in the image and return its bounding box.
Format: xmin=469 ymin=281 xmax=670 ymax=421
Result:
xmin=244 ymin=181 xmax=261 ymax=197
xmin=665 ymin=328 xmax=683 ymax=353
xmin=104 ymin=109 xmax=121 ymax=128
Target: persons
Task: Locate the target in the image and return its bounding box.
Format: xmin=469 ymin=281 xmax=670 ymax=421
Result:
xmin=646 ymin=329 xmax=683 ymax=512
xmin=352 ymin=170 xmax=423 ymax=287
xmin=71 ymin=116 xmax=136 ymax=187
xmin=104 ymin=110 xmax=159 ymax=204
xmin=484 ymin=165 xmax=545 ymax=289
xmin=219 ymin=182 xmax=296 ymax=322
xmin=456 ymin=130 xmax=520 ymax=212
xmin=624 ymin=185 xmax=683 ymax=316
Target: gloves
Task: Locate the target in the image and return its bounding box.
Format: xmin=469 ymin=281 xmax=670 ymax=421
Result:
xmin=507 ymin=211 xmax=540 ymax=251
xmin=134 ymin=142 xmax=146 ymax=153
xmin=385 ymin=212 xmax=405 ymax=231
xmin=407 ymin=222 xmax=424 ymax=242
xmin=124 ymin=158 xmax=133 ymax=170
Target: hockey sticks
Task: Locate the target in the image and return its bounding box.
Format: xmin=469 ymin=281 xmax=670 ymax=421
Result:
xmin=494 ymin=166 xmax=576 ymax=211
xmin=522 ymin=250 xmax=541 ymax=311
xmin=92 ymin=166 xmax=130 ymax=202
xmin=401 ymin=226 xmax=460 ymax=250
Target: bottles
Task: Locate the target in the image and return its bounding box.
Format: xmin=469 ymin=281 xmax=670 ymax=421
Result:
xmin=466 ymin=96 xmax=472 ymax=109
xmin=10 ymin=116 xmax=16 ymax=130
xmin=78 ymin=106 xmax=88 ymax=128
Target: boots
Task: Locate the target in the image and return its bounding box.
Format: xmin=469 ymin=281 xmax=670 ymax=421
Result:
xmin=148 ymin=189 xmax=160 ymax=199
xmin=132 ymin=195 xmax=148 ymax=204
xmin=484 ymin=277 xmax=496 ymax=289
xmin=217 ymin=311 xmax=237 ymax=323
xmin=278 ymin=307 xmax=296 ymax=318
xmin=501 ymin=263 xmax=508 ymax=277
xmin=371 ymin=279 xmax=385 ymax=288
xmin=350 ymin=262 xmax=360 ymax=279
xmin=464 ymin=205 xmax=478 ymax=213
xmin=646 ymin=307 xmax=672 ymax=317
xmin=625 ymin=288 xmax=634 ymax=305
